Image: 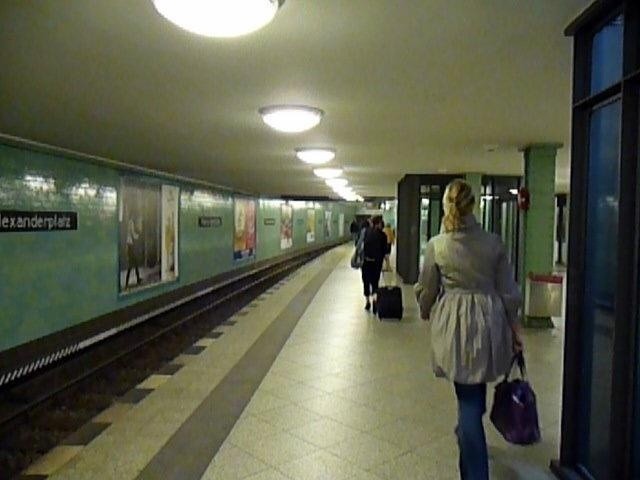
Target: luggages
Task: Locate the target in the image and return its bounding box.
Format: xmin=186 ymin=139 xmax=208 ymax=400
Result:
xmin=375 ymin=269 xmax=404 ymax=321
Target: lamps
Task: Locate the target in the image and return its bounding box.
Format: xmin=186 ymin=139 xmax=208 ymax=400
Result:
xmin=314 ymin=166 xmax=343 ymax=179
xmin=153 ymin=0 xmax=286 ymax=38
xmin=296 ymin=147 xmax=335 ymax=164
xmin=260 ymin=106 xmax=325 ymax=133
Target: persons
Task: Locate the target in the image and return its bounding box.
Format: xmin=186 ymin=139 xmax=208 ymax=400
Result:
xmin=415 ymin=175 xmax=523 ymax=480
xmin=126 ymin=210 xmax=142 ymax=287
xmin=350 ymin=219 xmax=358 ymax=241
xmin=382 ymin=224 xmax=394 ymax=272
xmin=362 ymin=214 xmax=388 ymax=315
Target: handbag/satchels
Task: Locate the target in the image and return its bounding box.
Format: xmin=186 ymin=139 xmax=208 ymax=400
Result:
xmin=489 ymin=350 xmax=541 ymax=445
xmin=351 ymin=228 xmax=366 ymax=268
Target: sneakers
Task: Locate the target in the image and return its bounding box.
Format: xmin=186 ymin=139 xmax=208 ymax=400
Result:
xmin=366 ymin=302 xmax=370 ymax=309
xmin=372 ymin=300 xmax=377 ymax=314
